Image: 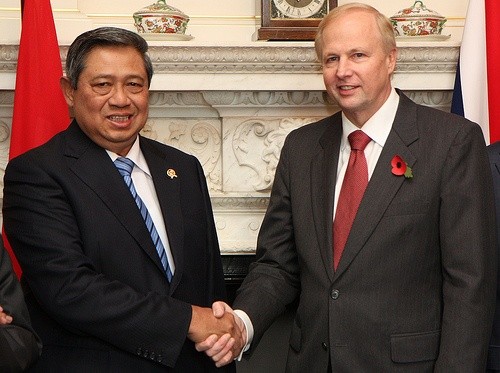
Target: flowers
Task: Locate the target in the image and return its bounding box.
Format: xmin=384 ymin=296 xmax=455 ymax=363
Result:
xmin=391 ymin=154 xmax=413 ymax=178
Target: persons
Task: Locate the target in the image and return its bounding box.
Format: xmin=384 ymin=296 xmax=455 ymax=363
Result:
xmin=0 ymin=25 xmax=246 ymax=372
xmin=194 ymin=2 xmax=500 ymax=373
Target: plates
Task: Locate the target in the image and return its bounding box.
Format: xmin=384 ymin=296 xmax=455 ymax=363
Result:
xmin=395 ymin=36 xmax=451 ymax=42
xmin=140 ymin=34 xmax=194 ymax=41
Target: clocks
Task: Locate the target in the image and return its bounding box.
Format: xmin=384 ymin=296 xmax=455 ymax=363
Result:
xmin=258 ymin=0 xmax=338 ymax=42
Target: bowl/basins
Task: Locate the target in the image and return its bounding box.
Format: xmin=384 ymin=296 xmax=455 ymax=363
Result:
xmin=133 ymin=0 xmax=190 ymax=34
xmin=389 ymin=0 xmax=447 ymax=37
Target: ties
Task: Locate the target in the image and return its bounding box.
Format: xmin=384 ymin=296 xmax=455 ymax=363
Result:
xmin=114 ymin=157 xmax=172 ymax=284
xmin=333 ymin=130 xmax=373 ymax=272
